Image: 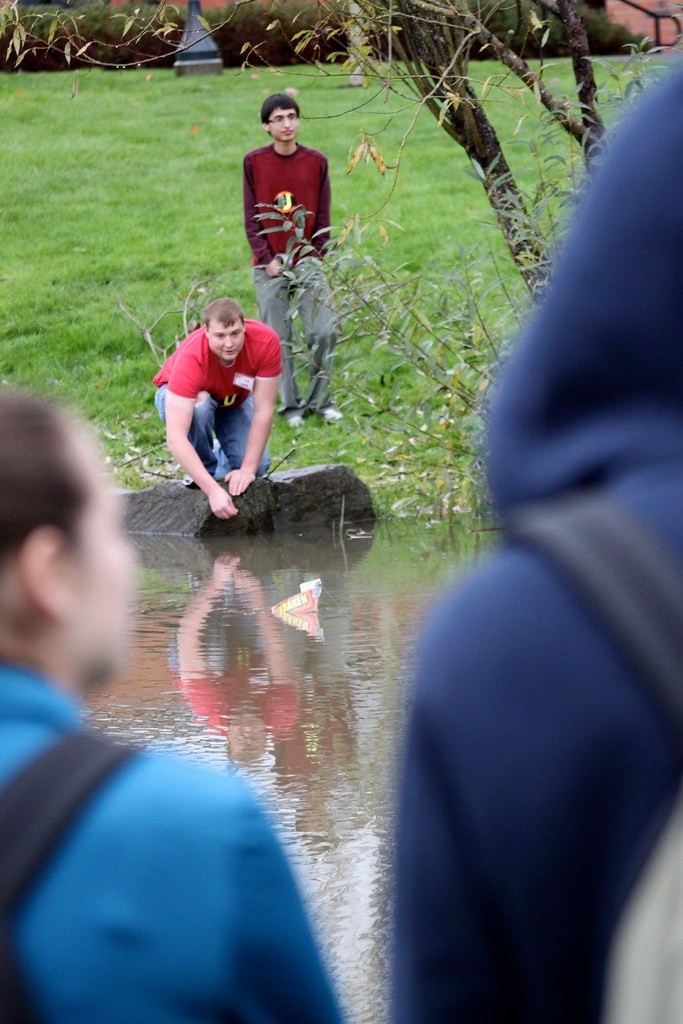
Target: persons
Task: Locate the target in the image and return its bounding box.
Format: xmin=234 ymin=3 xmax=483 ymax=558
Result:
xmin=242 ymin=93 xmax=349 ymax=424
xmin=152 ymin=298 xmax=283 ymax=518
xmin=392 ymin=49 xmax=683 ymax=1024
xmin=1 ymin=389 xmax=346 ymax=1024
xmin=167 ymin=560 xmax=309 ymax=760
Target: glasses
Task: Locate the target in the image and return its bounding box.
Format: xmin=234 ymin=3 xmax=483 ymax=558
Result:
xmin=266 ymin=112 xmax=298 ymax=124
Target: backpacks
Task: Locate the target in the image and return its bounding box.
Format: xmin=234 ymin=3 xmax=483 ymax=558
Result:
xmin=497 ymin=495 xmax=683 ymax=1024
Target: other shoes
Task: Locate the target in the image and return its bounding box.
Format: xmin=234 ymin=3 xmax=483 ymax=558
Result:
xmin=286 ymin=415 xmax=304 ymax=426
xmin=323 ymin=407 xmax=343 ymax=421
